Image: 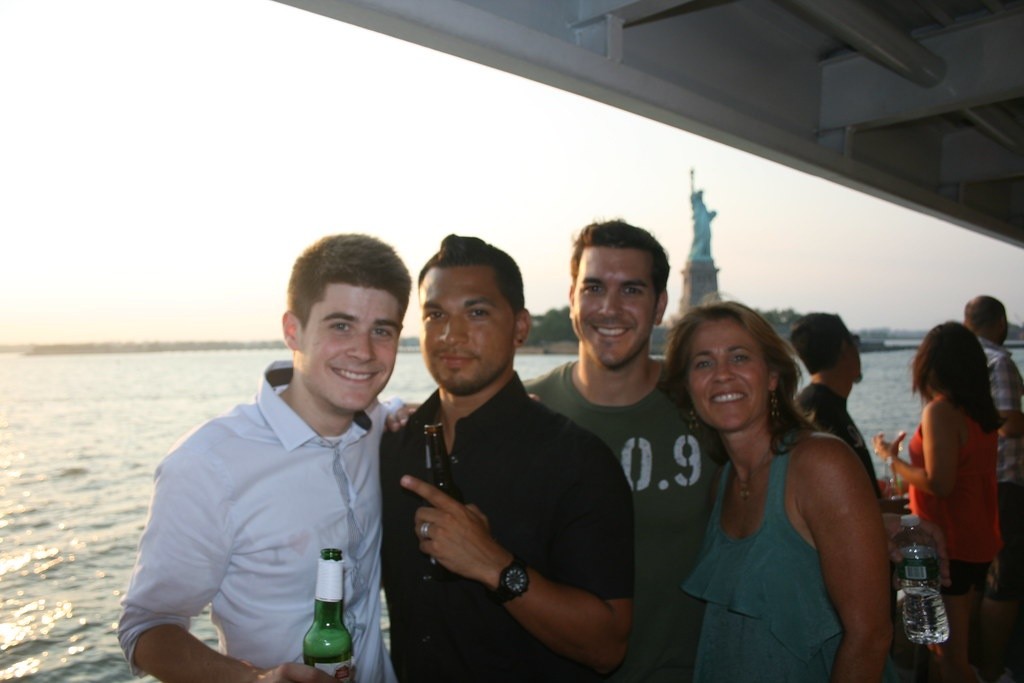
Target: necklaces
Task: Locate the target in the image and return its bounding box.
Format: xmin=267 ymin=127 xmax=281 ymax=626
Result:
xmin=737 ymin=450 xmax=772 ymax=500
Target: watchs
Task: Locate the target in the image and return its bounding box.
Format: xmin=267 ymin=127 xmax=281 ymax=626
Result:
xmin=491 ymin=552 xmax=529 ymax=604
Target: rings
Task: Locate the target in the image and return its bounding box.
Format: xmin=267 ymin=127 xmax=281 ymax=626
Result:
xmin=420 ymin=522 xmax=430 ymax=537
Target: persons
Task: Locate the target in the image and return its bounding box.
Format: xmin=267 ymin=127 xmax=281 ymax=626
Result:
xmin=688 ymin=170 xmax=717 ymax=260
xmin=792 ymin=312 xmax=896 ymax=651
xmin=379 ymin=234 xmax=635 ymax=683
xmin=963 ymin=297 xmax=1024 ymax=683
xmin=874 ymin=321 xmax=1004 ymax=683
xmin=116 ymin=234 xmax=541 ymax=683
xmin=383 ymin=220 xmax=949 ymax=683
xmin=662 ymin=301 xmax=903 ymax=683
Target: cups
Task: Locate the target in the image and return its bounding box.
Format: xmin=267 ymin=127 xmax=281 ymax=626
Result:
xmin=885 ymin=457 xmax=902 ymax=500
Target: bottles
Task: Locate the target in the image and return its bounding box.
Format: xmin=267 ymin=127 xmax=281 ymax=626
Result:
xmin=421 ymin=421 xmax=466 ymax=581
xmin=303 ymin=548 xmax=354 ymax=683
xmin=896 ymin=514 xmax=950 ymax=645
